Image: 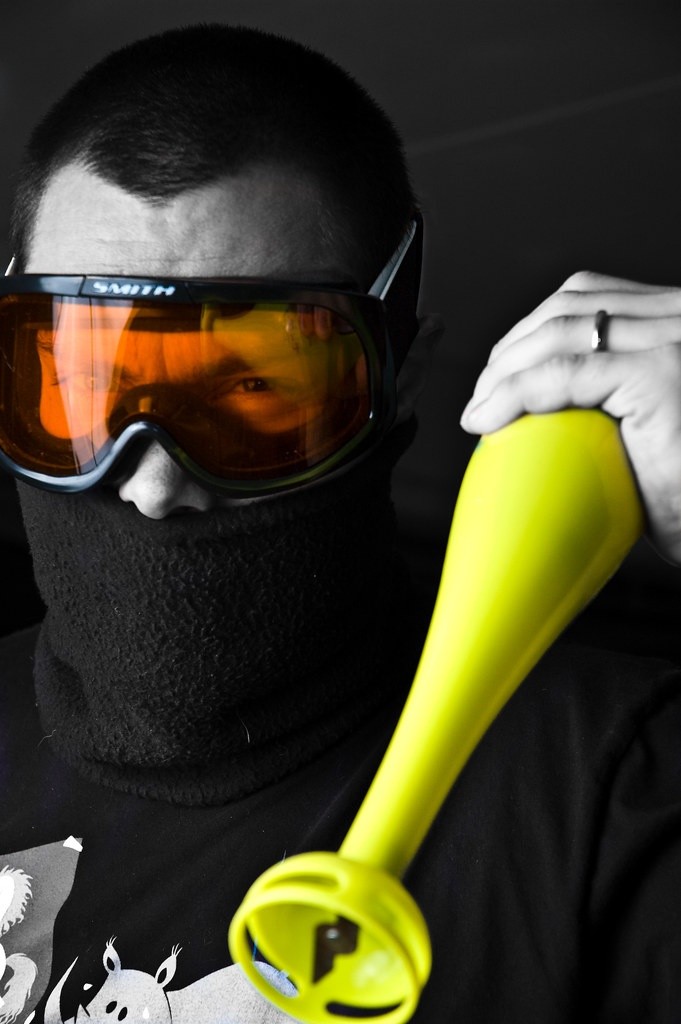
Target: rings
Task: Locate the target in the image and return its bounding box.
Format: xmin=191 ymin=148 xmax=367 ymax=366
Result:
xmin=596 ymin=310 xmax=609 ymax=352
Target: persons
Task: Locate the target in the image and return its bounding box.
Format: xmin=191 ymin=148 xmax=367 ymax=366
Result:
xmin=0 ymin=21 xmax=681 ymax=1024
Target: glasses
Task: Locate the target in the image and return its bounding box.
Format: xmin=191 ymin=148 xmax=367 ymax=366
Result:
xmin=1 ymin=221 xmax=427 ymax=503
